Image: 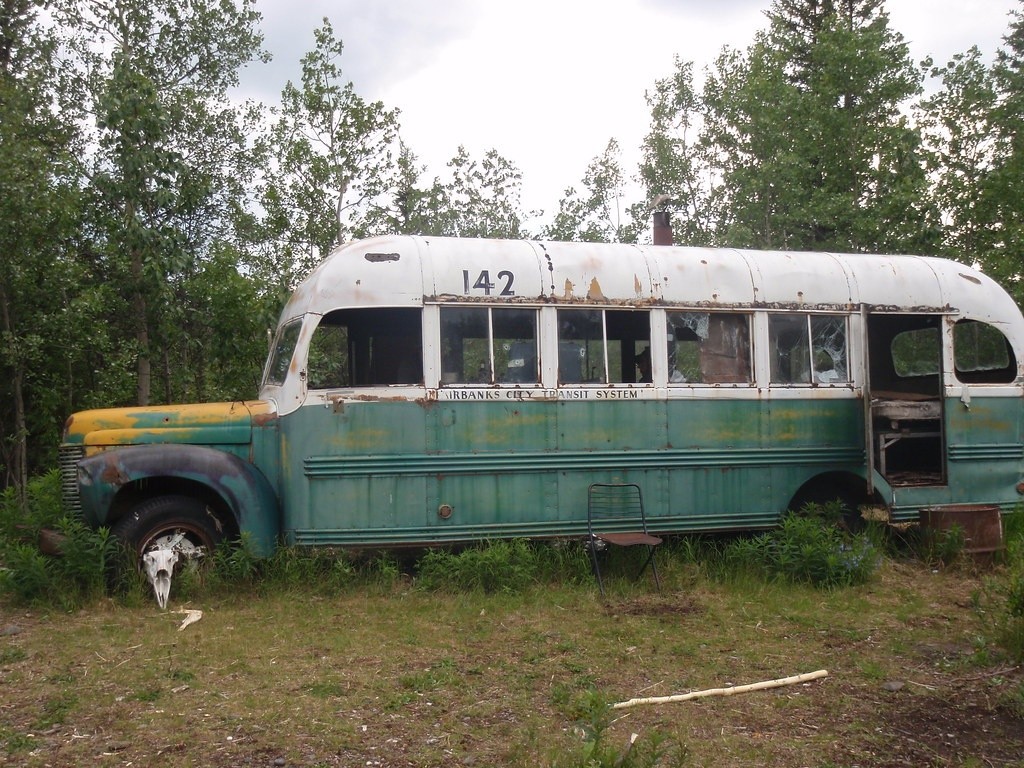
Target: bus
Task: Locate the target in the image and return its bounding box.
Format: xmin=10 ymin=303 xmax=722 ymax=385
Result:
xmin=57 ymin=206 xmax=1024 ymax=598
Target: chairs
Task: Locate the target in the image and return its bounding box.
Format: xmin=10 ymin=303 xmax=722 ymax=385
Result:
xmin=588 ymin=482 xmax=663 ymax=597
